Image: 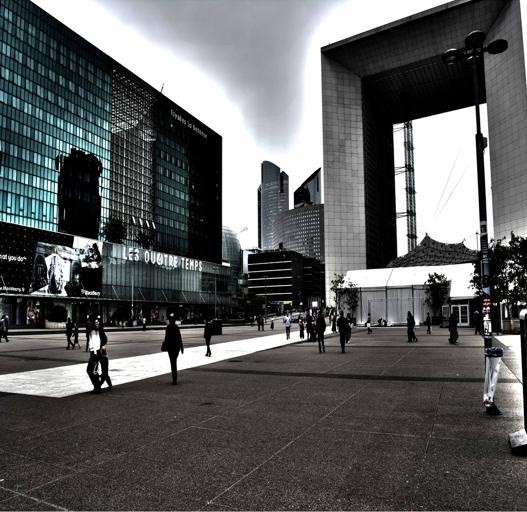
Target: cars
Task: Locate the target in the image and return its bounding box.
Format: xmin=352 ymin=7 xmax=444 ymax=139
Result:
xmin=291 ymin=315 xmax=306 ymax=323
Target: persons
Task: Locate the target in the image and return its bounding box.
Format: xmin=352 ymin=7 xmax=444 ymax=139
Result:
xmin=1 ymin=315 xmax=10 ymax=343
xmin=448 ymin=310 xmax=480 ymax=345
xmin=162 ymin=312 xmax=185 ymax=385
xmin=406 ymin=310 xmax=419 ymax=343
xmin=42 ymin=242 xmax=101 ymax=296
xmin=256 ymin=312 xmax=274 ymax=331
xmin=425 ymin=313 xmax=432 ymax=335
xmin=282 ymin=308 xmax=373 ymax=354
xmin=203 ymin=318 xmax=212 ymax=357
xmin=141 ymin=317 xmax=146 ymax=329
xmin=66 ymin=315 xmax=113 ymax=393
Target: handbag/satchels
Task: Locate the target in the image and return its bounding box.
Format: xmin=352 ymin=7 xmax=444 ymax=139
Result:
xmin=160 ymin=341 xmax=168 ymax=352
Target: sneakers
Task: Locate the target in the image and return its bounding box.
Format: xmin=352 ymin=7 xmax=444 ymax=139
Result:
xmin=318 ymin=350 xmax=346 ymax=354
xmin=65 ymin=346 xmax=82 ymax=350
xmin=92 ymin=384 xmax=113 ymax=394
xmin=205 ymin=352 xmax=211 ymax=357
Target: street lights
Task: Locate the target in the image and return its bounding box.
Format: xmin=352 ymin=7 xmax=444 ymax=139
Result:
xmin=129 ymin=251 xmax=138 ymax=318
xmin=213 ymin=267 xmax=219 ymax=318
xmin=443 ymin=29 xmax=508 ymax=348
